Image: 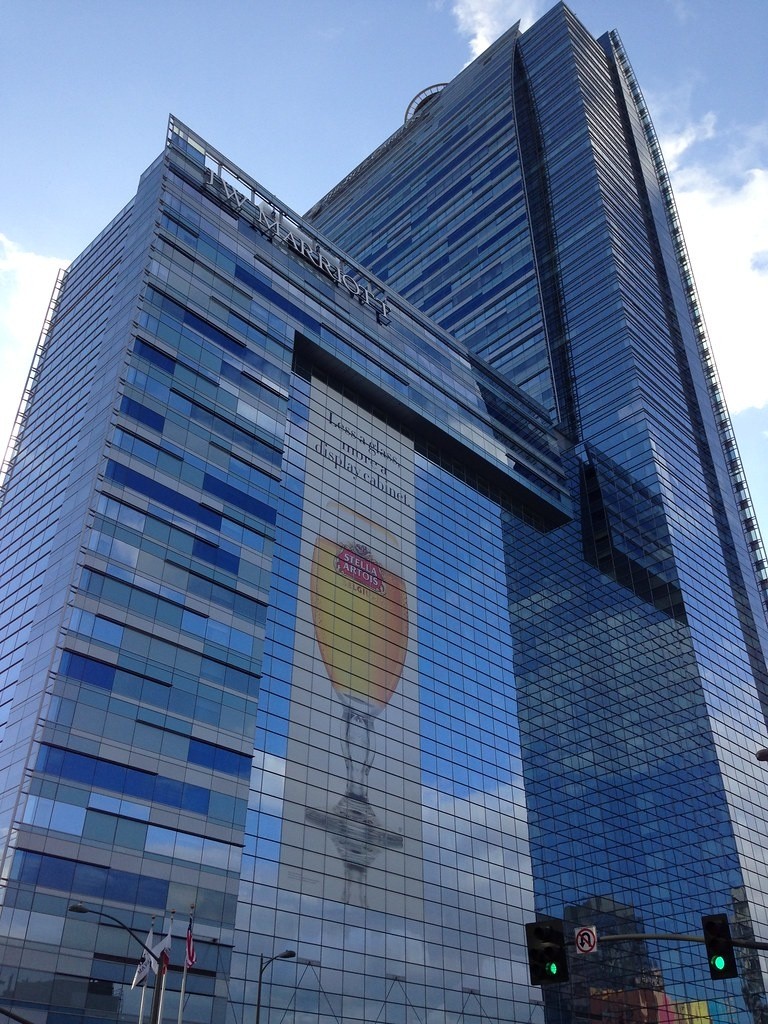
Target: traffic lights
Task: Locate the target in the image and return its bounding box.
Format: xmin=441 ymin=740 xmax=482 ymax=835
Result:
xmin=702 ymin=913 xmax=738 ymax=980
xmin=525 ymin=919 xmax=569 ymax=985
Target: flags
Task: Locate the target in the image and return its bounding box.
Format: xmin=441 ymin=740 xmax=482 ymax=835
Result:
xmin=130 ymin=927 xmax=153 ymax=990
xmin=148 ymin=924 xmax=171 ymax=975
xmin=186 ymin=915 xmax=198 ymax=968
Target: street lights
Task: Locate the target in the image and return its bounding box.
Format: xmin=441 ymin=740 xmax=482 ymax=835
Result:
xmin=255 ymin=950 xmax=295 ymax=1023
xmin=67 ymin=903 xmax=163 ymax=1024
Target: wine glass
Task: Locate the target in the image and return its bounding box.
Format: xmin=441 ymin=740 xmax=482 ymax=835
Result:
xmin=306 ymin=501 xmax=411 ymax=851
